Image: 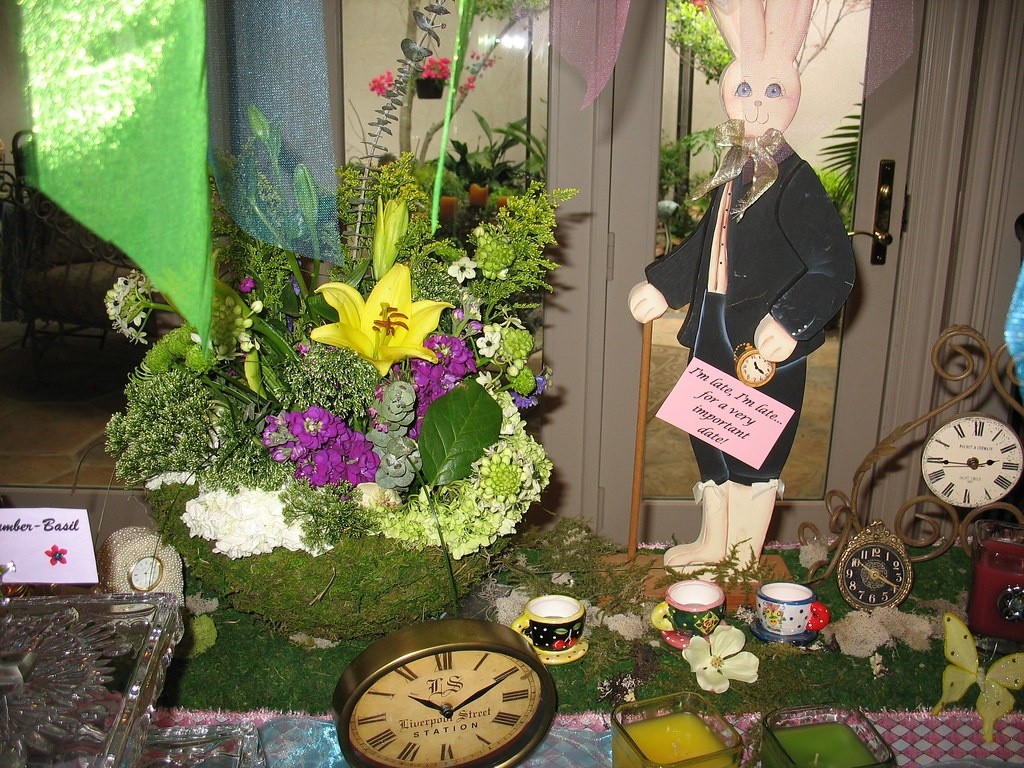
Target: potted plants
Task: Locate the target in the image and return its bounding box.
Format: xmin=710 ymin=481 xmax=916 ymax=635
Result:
xmin=430 ymin=109 xmax=530 ymax=221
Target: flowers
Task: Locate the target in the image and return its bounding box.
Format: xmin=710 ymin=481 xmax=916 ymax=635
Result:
xmin=681 ymin=624 xmax=758 ymax=693
xmin=370 ymin=47 xmax=503 ymax=100
xmin=106 ymin=0 xmax=583 ymax=562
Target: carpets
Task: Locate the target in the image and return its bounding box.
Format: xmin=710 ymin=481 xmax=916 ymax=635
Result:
xmin=645 ymin=343 xmax=690 ymax=427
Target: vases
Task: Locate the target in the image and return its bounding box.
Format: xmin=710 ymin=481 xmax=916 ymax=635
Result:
xmin=415 ymin=78 xmax=443 ymax=99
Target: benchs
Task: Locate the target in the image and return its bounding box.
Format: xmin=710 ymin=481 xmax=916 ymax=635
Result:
xmin=0 ymin=126 xmax=242 ymax=356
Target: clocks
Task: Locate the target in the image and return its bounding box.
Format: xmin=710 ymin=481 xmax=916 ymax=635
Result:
xmin=88 ymin=528 xmax=186 ymax=612
xmin=919 ymin=412 xmax=1024 ymax=508
xmin=734 ymin=344 xmax=775 ymax=387
xmin=838 ymin=520 xmax=913 ymax=613
xmin=332 ymin=619 xmax=556 ymax=768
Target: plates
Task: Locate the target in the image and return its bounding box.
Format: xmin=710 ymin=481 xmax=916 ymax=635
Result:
xmin=661 ymin=619 xmax=728 ymax=649
xmin=651 ymin=599 xmax=674 ymax=631
xmin=510 ymin=615 xmax=589 ymax=664
xmin=749 ymin=618 xmax=818 ymax=646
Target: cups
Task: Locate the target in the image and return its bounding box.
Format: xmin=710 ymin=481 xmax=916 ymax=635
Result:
xmin=651 ymin=580 xmax=727 ymax=639
xmin=756 ymin=581 xmax=830 ymax=636
xmin=510 ymin=595 xmax=587 ymax=654
